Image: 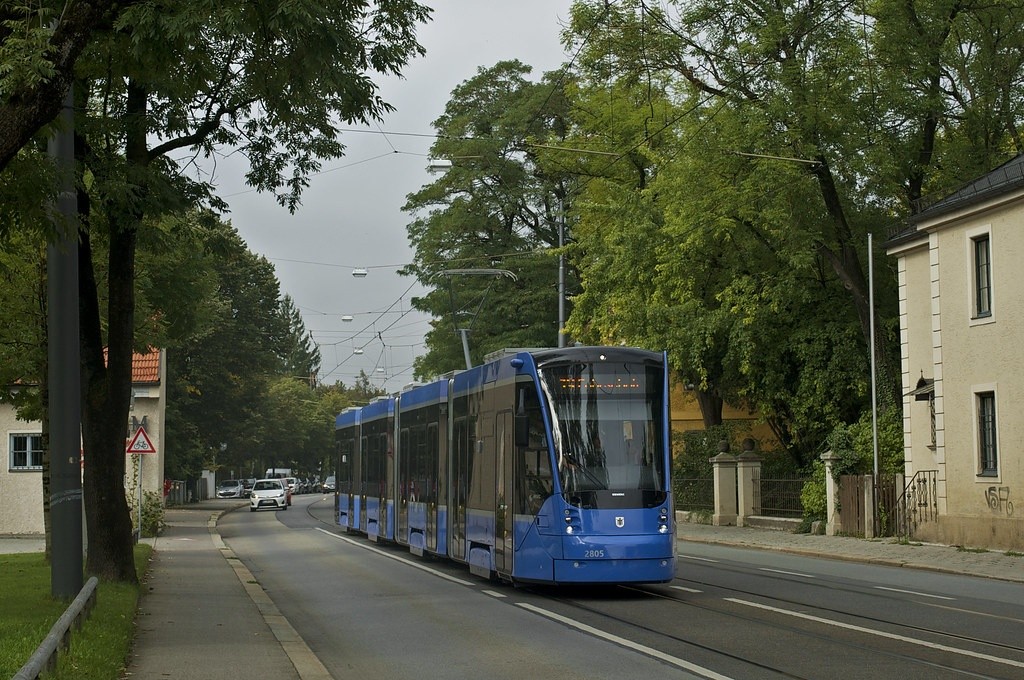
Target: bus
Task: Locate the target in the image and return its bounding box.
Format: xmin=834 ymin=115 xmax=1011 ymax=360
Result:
xmin=335 ymin=269 xmax=676 ymax=594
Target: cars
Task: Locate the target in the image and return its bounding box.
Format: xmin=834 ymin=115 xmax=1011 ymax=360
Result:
xmin=244 ymin=476 xmax=325 ymax=498
xmin=216 ymin=479 xmax=245 ymax=499
xmin=246 ymin=478 xmax=290 ymax=511
xmin=323 ymin=475 xmax=337 ymax=494
